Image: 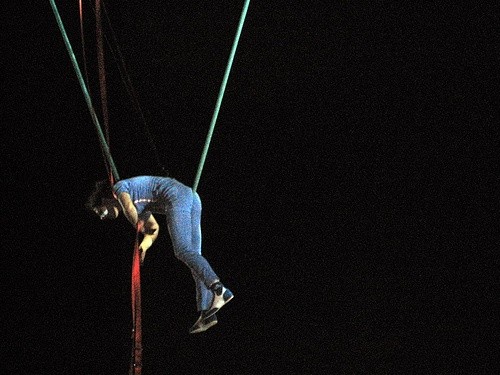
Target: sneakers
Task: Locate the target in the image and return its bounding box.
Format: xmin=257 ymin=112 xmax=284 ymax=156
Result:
xmin=190 ymin=312 xmax=218 ymax=333
xmin=203 ymin=283 xmax=234 ymax=319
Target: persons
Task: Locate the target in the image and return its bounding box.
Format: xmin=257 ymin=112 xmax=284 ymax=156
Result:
xmin=89 ymin=175 xmax=235 ymax=334
